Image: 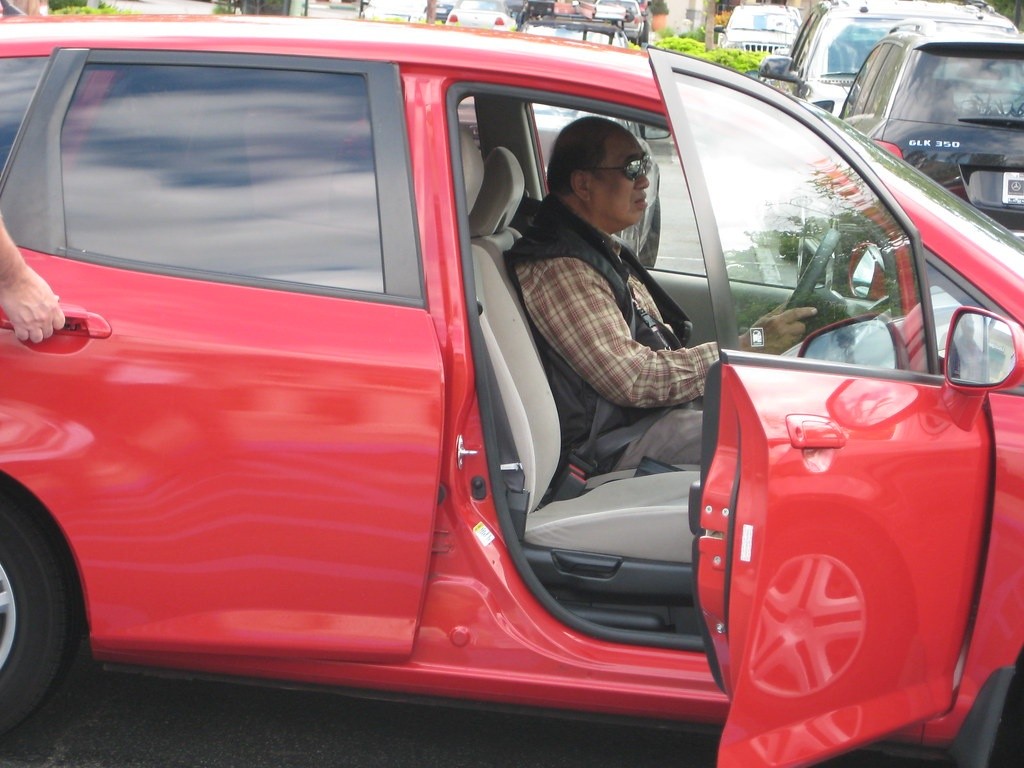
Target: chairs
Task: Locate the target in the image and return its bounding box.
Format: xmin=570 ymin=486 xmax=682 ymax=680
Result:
xmin=460 ymin=128 xmax=701 ymax=596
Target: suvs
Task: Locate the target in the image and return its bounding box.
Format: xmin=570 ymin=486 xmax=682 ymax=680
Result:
xmin=715 ymin=3 xmax=803 ymax=55
xmin=759 ymin=0 xmax=1020 ymax=122
xmin=517 ymin=11 xmax=630 ymax=51
xmin=797 ymin=19 xmax=1024 ymax=283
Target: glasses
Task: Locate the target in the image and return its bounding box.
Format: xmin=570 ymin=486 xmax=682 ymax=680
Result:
xmin=581 ymin=152 xmax=651 ymax=182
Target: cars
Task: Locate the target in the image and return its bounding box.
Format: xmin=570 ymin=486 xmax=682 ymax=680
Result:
xmin=574 ymin=0 xmax=646 ymax=42
xmin=0 ymin=9 xmax=1024 ymax=768
xmin=435 ymin=0 xmax=522 ymax=31
xmin=362 ymin=0 xmax=428 ymax=21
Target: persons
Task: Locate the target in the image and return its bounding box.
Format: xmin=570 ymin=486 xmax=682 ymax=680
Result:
xmin=0 ymin=214 xmax=65 ymax=343
xmin=510 ymin=116 xmax=818 ymax=467
